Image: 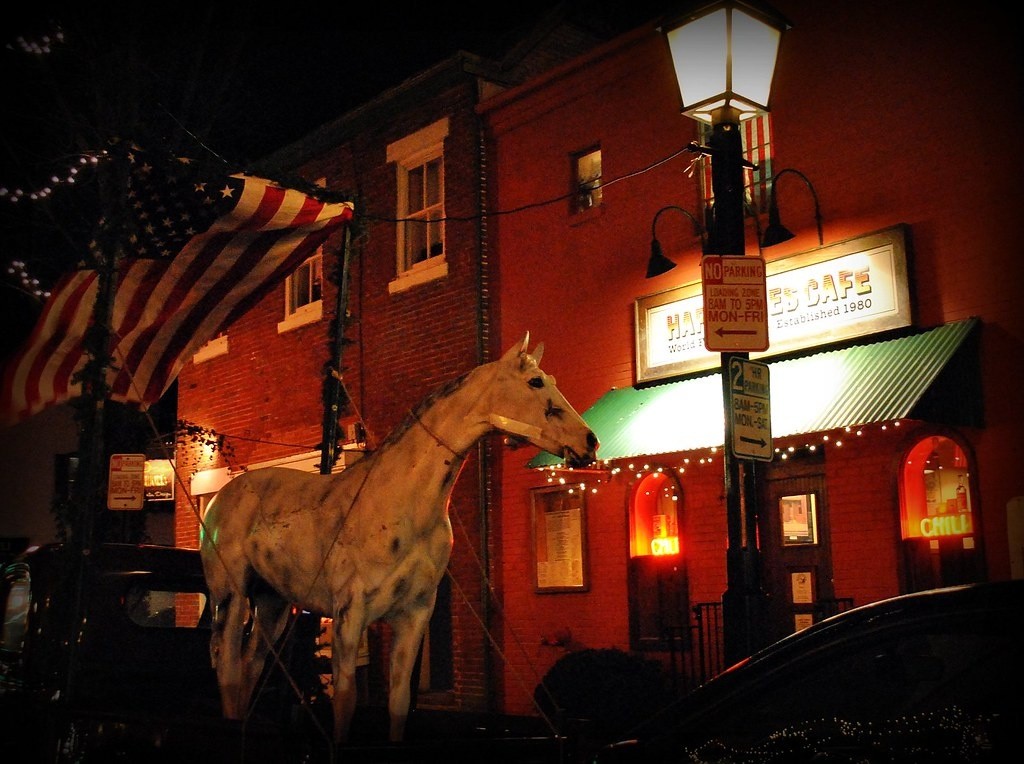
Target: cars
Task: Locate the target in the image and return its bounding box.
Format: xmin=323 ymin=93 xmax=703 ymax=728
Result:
xmin=588 ymin=579 xmax=1024 ymax=764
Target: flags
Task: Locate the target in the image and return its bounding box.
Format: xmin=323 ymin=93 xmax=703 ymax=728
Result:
xmin=0 ymin=0 xmax=354 ymax=433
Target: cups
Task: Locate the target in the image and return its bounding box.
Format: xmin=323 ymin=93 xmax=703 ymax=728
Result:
xmin=946 ymin=499 xmax=956 ymax=513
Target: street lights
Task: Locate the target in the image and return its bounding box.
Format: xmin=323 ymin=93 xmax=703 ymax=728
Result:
xmin=656 ymin=1 xmax=793 ymax=665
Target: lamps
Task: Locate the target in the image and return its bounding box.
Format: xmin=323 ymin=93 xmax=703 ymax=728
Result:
xmin=644 ymin=205 xmax=706 ymax=280
xmin=759 ymin=168 xmax=826 ymax=253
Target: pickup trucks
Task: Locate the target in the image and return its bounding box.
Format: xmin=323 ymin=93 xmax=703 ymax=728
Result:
xmin=0 ymin=537 xmax=570 ymax=764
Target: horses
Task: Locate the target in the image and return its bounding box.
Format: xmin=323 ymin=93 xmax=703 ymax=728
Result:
xmin=199 ymin=332 xmax=600 ymax=725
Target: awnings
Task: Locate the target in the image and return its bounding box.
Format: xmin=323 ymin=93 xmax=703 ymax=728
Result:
xmin=523 ymin=313 xmax=985 ymax=470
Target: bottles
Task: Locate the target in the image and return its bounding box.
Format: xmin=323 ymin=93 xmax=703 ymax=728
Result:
xmin=956 ymin=475 xmax=968 ymax=511
